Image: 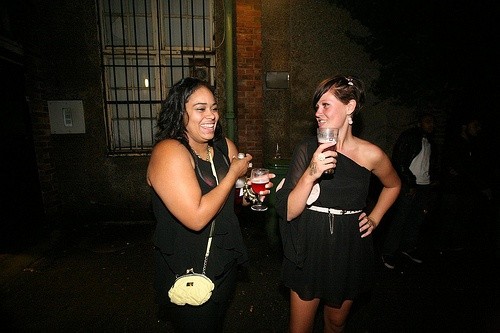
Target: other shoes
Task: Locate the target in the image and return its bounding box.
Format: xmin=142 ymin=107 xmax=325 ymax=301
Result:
xmin=402 ymin=246 xmax=423 ymax=264
xmin=381 ymin=255 xmax=395 ymax=269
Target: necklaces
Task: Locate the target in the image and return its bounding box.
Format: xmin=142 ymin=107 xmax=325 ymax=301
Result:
xmin=189 ymin=144 xmax=210 ymax=162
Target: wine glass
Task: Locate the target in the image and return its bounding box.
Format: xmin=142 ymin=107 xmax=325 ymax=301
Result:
xmin=251 ymin=168 xmax=269 ymax=211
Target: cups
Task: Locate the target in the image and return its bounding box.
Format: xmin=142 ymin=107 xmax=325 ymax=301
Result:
xmin=317 ymin=128 xmax=339 ymax=174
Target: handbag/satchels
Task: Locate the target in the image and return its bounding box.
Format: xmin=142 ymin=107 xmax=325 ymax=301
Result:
xmin=167 ymin=268 xmax=215 ymax=306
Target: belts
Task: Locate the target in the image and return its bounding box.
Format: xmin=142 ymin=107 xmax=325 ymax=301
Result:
xmin=306 ymin=205 xmax=363 ymax=215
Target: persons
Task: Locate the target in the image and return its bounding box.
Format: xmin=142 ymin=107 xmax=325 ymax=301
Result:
xmin=381 ymin=113 xmax=443 ymax=269
xmin=437 ymin=115 xmax=495 ymax=257
xmin=143 ymin=77 xmax=276 ymax=333
xmin=271 ymin=73 xmax=401 ymax=333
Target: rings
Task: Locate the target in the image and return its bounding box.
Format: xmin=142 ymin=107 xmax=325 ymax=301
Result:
xmin=317 ymin=154 xmax=325 ymax=161
